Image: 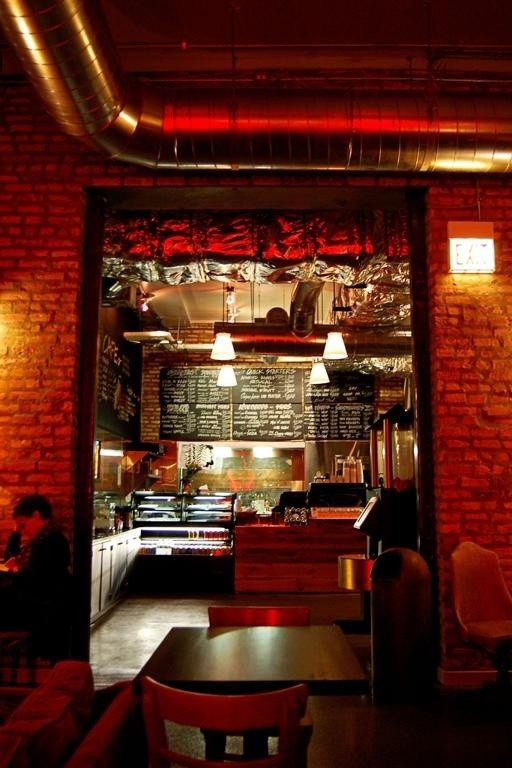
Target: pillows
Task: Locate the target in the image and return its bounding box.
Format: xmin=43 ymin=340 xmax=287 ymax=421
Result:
xmin=0 ymin=659 xmax=96 ymax=767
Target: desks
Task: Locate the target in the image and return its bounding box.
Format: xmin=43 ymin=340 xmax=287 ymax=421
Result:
xmin=134 ymin=626 xmax=368 ymax=759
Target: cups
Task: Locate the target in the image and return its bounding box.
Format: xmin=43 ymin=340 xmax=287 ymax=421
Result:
xmin=314 ymin=455 xmax=364 ymax=483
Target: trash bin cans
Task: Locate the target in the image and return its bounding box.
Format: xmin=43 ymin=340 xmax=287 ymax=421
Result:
xmin=370 ymin=548 xmax=433 ymax=706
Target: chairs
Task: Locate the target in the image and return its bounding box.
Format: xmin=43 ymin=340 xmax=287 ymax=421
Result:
xmin=0 ymin=682 xmax=136 ymax=768
xmin=450 ymin=542 xmax=512 ymax=654
xmin=140 ymin=676 xmax=313 ymax=767
xmin=201 ymin=606 xmax=312 ymax=767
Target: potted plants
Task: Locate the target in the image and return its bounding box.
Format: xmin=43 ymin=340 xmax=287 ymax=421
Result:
xmin=180 ymin=444 xmax=214 ymax=494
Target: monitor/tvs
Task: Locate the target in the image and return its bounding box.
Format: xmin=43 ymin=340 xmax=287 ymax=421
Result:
xmin=365 ymin=488 xmax=383 ymax=507
xmin=352 ymin=496 xmax=381 ymax=530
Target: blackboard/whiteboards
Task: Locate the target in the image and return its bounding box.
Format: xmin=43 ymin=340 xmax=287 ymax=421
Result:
xmin=158 ymin=366 xmax=378 ymax=443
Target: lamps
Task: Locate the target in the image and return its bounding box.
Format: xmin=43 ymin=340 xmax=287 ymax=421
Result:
xmin=309 ymin=294 xmax=331 ymax=385
xmin=136 ymin=293 xmax=155 ymax=311
xmin=447 ymin=221 xmax=496 ymax=274
xmin=210 ymin=282 xmax=240 ymax=387
xmin=324 ymin=280 xmax=349 ymax=359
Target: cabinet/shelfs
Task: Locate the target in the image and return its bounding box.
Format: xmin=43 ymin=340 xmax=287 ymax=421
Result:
xmin=90 ymin=528 xmax=142 ymax=624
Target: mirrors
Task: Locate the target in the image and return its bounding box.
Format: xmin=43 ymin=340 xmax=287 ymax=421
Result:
xmin=93 ymin=425 xmax=145 ymax=536
xmin=178 ymin=447 xmax=306 ymax=507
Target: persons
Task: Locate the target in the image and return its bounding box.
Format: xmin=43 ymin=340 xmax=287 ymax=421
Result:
xmin=4 ymin=494 xmax=70 ymax=634
